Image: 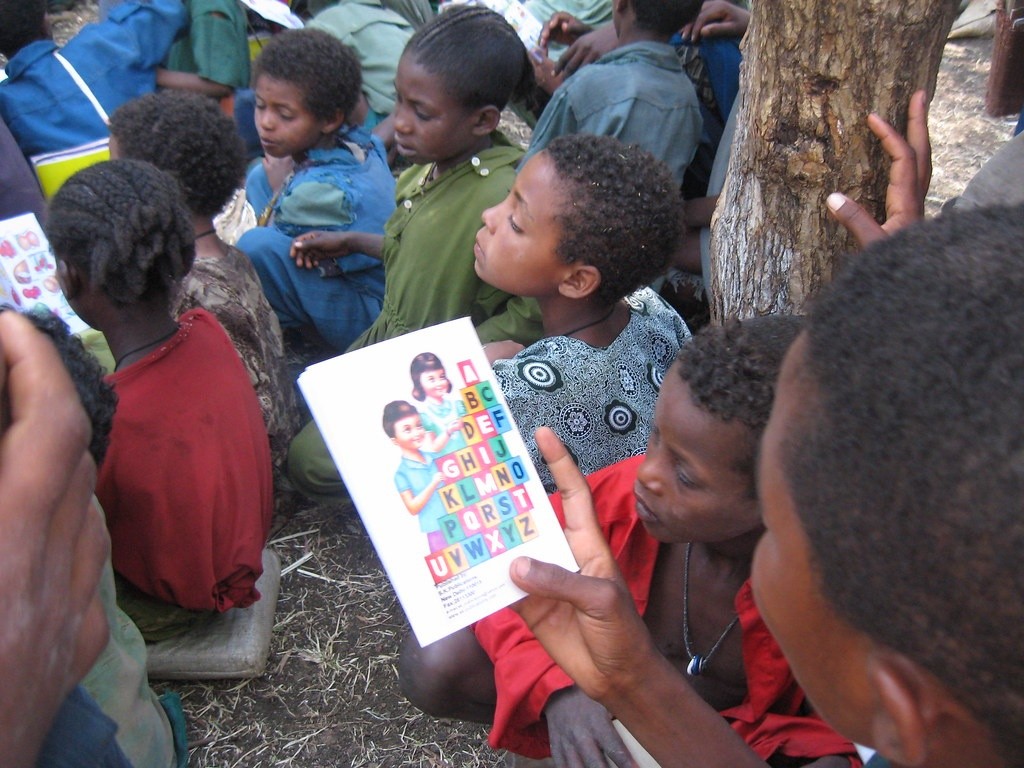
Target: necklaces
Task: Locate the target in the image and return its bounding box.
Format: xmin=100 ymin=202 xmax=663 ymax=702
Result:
xmin=114 ymin=325 xmax=180 ymax=371
xmin=428 ymin=164 xmax=438 ymax=182
xmin=683 ymin=542 xmax=740 ymax=677
xmin=195 ymin=228 xmax=217 ymax=240
xmin=562 ymin=302 xmax=616 ymax=337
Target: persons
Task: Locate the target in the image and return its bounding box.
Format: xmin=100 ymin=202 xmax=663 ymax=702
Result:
xmin=401 ymin=313 xmax=865 ymax=768
xmin=509 ymin=200 xmax=1024 ymax=768
xmin=0 ymin=0 xmax=1024 ymax=636
xmin=0 ymin=299 xmax=193 ymax=768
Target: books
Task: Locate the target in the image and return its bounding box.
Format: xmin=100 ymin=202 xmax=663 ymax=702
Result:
xmin=296 ymin=317 xmax=580 ymax=648
xmin=0 ymin=211 xmax=91 ymax=336
